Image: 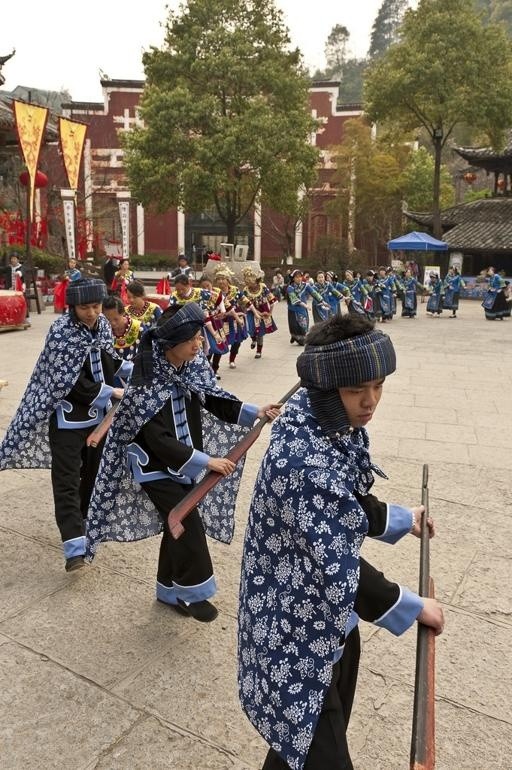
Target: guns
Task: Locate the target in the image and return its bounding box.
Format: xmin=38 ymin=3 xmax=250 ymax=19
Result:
xmin=168 ymin=381 xmax=300 ymax=538
xmin=410 ymin=464 xmax=435 ymax=770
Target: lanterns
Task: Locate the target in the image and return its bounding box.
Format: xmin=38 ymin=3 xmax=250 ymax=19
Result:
xmin=464 ymin=171 xmax=476 ymax=186
xmin=496 ymin=179 xmax=508 ymax=191
xmin=19 ymin=169 xmax=50 ymax=191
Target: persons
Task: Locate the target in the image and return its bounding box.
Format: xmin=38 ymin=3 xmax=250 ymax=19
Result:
xmin=270 ymin=267 xmax=426 ymax=346
xmin=502 ymin=280 xmax=512 ymax=305
xmin=103 ymin=256 xmax=119 ymax=296
xmin=84 ymin=301 xmax=285 ymax=623
xmin=168 ymin=255 xmax=193 ymax=287
xmin=1 ymin=276 xmax=133 ymax=572
xmin=64 ymin=257 xmax=82 ymax=313
xmin=444 ymin=266 xmax=467 ymax=318
xmin=103 ymin=264 xmax=277 ymax=381
xmin=482 ymin=266 xmax=512 ymax=320
xmin=7 ymin=252 xmax=30 ymax=318
xmin=112 ymin=259 xmax=136 ymax=305
xmin=207 ymin=250 xmax=221 ymax=260
xmin=238 ymin=314 xmax=444 ymax=770
xmin=426 ymin=270 xmax=445 ymax=317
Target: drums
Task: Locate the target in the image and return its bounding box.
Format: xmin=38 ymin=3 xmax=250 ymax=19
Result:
xmin=0 ymin=290 xmax=26 ymax=327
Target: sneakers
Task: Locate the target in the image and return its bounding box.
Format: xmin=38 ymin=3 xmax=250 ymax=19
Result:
xmin=157 ymin=596 xmax=217 ymax=622
xmin=65 ymin=555 xmax=84 ymax=572
